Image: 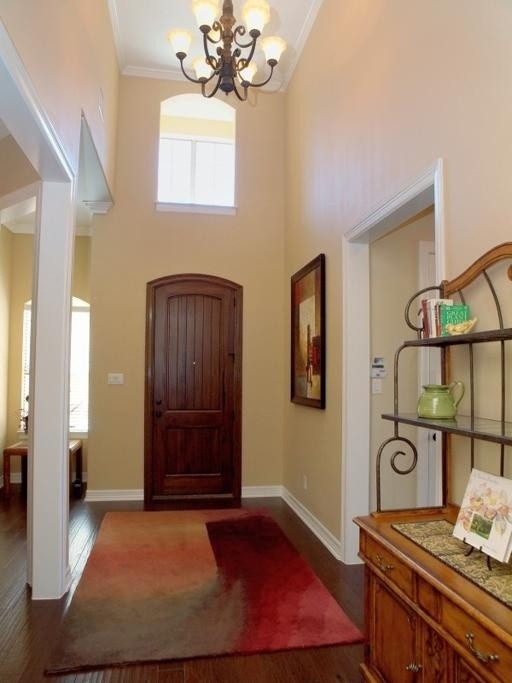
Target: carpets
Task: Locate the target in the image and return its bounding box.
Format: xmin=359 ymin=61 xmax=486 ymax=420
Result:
xmin=42 ymin=503 xmax=366 ymax=677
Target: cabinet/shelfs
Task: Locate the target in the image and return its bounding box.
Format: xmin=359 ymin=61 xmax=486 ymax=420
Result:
xmin=354 ymin=517 xmax=511 ymax=681
xmin=375 ymin=243 xmax=510 ymax=513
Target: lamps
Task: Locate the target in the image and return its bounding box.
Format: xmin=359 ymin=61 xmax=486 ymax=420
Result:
xmin=168 ymin=0 xmax=287 ymax=102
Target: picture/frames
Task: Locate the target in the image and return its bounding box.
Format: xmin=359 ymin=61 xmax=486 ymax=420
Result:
xmin=288 ymin=251 xmax=326 ymax=409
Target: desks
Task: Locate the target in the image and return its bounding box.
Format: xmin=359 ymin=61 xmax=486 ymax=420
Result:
xmin=2 ymin=436 xmax=85 ymax=501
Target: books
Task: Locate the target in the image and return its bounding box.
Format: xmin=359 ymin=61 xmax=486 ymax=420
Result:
xmin=422 ymin=297 xmax=471 ymax=339
